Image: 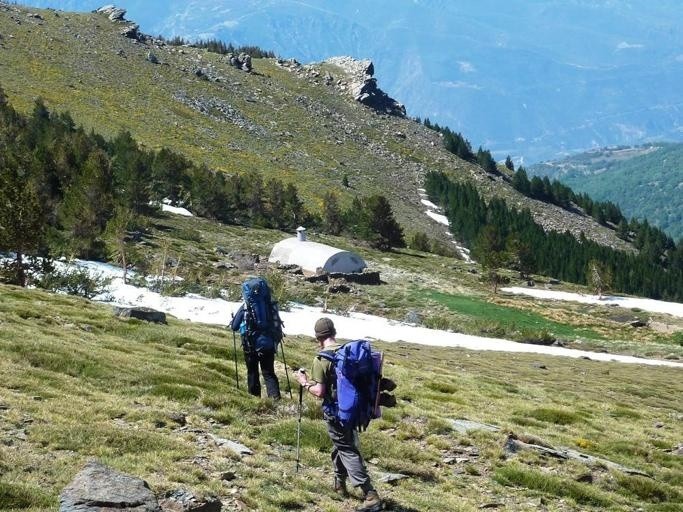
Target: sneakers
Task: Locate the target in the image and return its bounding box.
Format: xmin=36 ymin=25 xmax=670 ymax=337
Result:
xmin=332 ymin=477 xmax=348 ymax=496
xmin=356 ymin=490 xmax=383 ymax=512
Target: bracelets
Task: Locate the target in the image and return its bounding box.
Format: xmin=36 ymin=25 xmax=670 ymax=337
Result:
xmin=306 ymin=384 xmax=314 ymax=392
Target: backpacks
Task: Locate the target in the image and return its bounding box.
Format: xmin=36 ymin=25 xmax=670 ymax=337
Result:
xmin=318 ymin=340 xmax=383 ymax=428
xmin=240 ymin=279 xmax=282 ymax=365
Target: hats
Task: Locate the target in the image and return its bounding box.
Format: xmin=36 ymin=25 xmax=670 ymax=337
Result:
xmin=314 ymin=318 xmax=336 ymax=338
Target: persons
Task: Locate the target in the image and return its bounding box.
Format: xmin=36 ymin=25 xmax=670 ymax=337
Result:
xmin=232 ymin=287 xmax=282 ymax=400
xmin=291 ymin=317 xmax=384 ymax=512
